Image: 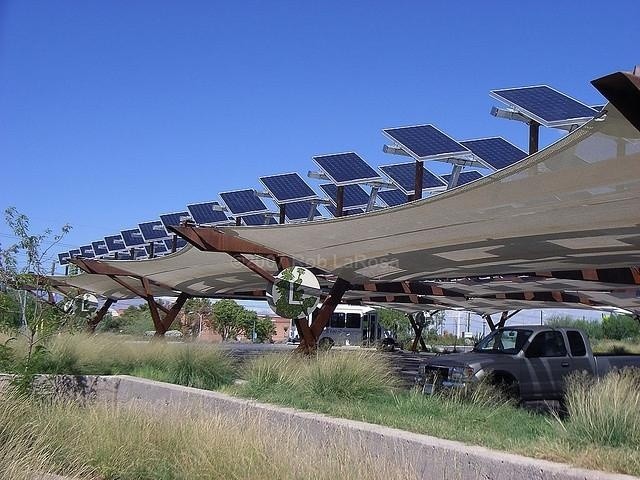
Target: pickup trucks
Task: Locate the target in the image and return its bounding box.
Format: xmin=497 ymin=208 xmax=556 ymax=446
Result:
xmin=416 ymin=325 xmax=640 ymax=411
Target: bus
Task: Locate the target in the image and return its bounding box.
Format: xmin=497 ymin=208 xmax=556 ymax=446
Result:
xmin=288 ymin=302 xmax=398 ymax=353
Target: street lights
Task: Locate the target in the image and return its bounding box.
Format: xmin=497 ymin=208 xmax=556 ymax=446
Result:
xmin=188 ymin=311 xmax=211 ymax=338
